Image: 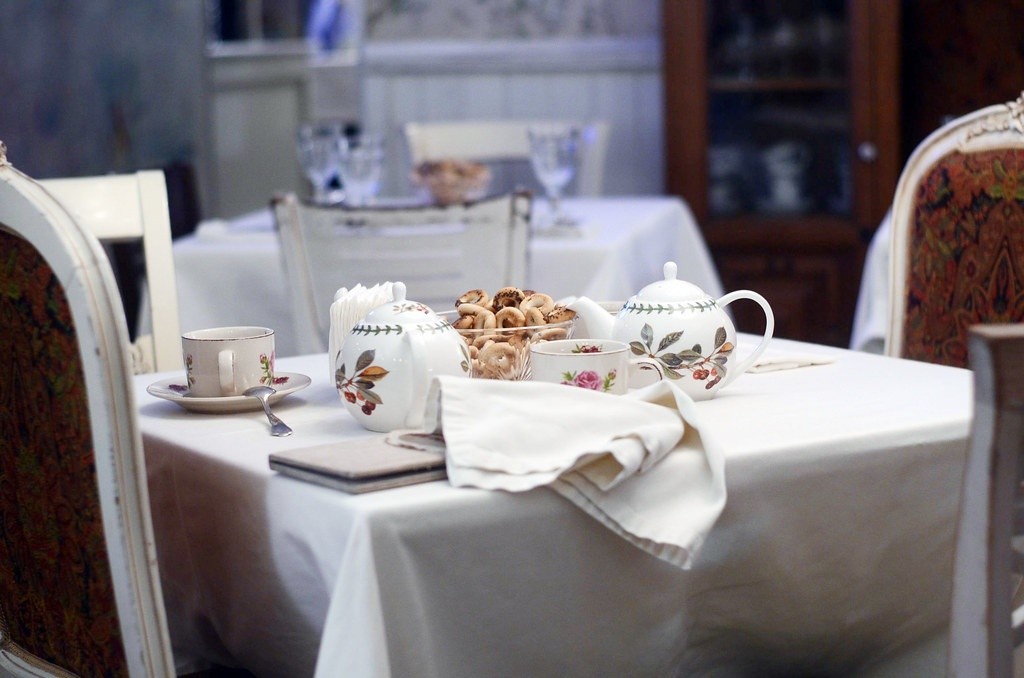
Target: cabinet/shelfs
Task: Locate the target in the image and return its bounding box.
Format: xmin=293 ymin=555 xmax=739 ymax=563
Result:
xmin=658 ymin=0 xmax=1024 ymax=347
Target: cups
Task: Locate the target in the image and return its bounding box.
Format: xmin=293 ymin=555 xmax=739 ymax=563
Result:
xmin=180 ymin=326 xmax=275 ymax=397
xmin=530 ymin=339 xmax=663 ymax=397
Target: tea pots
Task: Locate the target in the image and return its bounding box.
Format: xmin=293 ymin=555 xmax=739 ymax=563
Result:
xmin=566 ymin=262 xmax=775 ymax=401
xmin=334 ymin=281 xmax=472 ymax=433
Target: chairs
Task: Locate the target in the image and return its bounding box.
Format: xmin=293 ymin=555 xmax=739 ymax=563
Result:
xmin=404 ymin=118 xmax=614 ymax=198
xmin=269 ymin=189 xmax=533 ymax=353
xmin=0 ymin=135 xmax=225 ymax=678
xmin=881 ymin=93 xmax=1024 ymax=678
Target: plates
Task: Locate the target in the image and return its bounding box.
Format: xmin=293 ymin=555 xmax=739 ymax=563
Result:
xmin=146 ymin=372 xmax=310 ymax=413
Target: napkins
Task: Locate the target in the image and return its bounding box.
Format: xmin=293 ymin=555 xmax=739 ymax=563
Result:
xmin=197 ymin=218 xmax=276 ymax=242
xmin=389 ymin=374 xmax=728 ymax=571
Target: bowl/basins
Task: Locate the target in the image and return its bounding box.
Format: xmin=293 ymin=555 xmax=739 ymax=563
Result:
xmin=435 ymin=309 xmax=581 ymax=382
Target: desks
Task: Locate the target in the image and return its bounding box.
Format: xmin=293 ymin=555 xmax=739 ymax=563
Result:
xmin=136 ymin=196 xmax=736 ymax=358
xmin=129 ymin=331 xmax=973 ymax=678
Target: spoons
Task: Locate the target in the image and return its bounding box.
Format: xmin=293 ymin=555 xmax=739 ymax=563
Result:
xmin=243 ymin=386 xmax=292 ymax=437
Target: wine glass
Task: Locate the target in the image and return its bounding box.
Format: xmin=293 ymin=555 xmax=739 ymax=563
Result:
xmin=296 ymin=124 xmax=384 ymax=228
xmin=528 ymin=126 xmax=582 ymax=223
